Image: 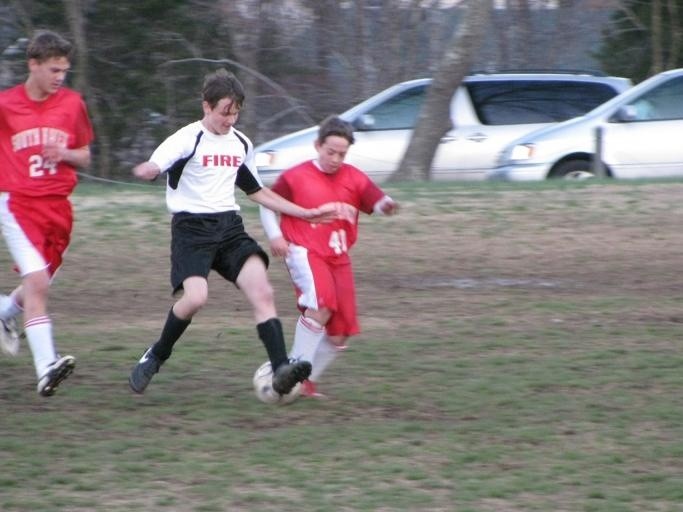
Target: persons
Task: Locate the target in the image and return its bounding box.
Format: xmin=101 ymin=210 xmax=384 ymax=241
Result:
xmin=259 ymin=114 xmax=400 ymax=399
xmin=129 ymin=69 xmax=339 ymax=394
xmin=0 ymin=29 xmax=94 ymax=399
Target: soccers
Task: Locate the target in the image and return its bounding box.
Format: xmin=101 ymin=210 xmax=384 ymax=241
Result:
xmin=253 ymin=359 xmax=301 ymax=404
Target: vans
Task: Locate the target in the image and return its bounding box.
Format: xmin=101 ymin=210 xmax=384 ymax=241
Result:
xmin=245 ymin=64 xmax=640 ymax=186
xmin=488 ymin=61 xmax=683 ymax=187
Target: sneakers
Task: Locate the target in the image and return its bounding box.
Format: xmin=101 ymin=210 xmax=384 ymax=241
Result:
xmin=36 ymin=355 xmax=75 ymax=396
xmin=272 ymin=358 xmax=320 ymax=396
xmin=0 ymin=295 xmax=21 ymax=355
xmin=128 ymin=348 xmax=158 ymax=393
